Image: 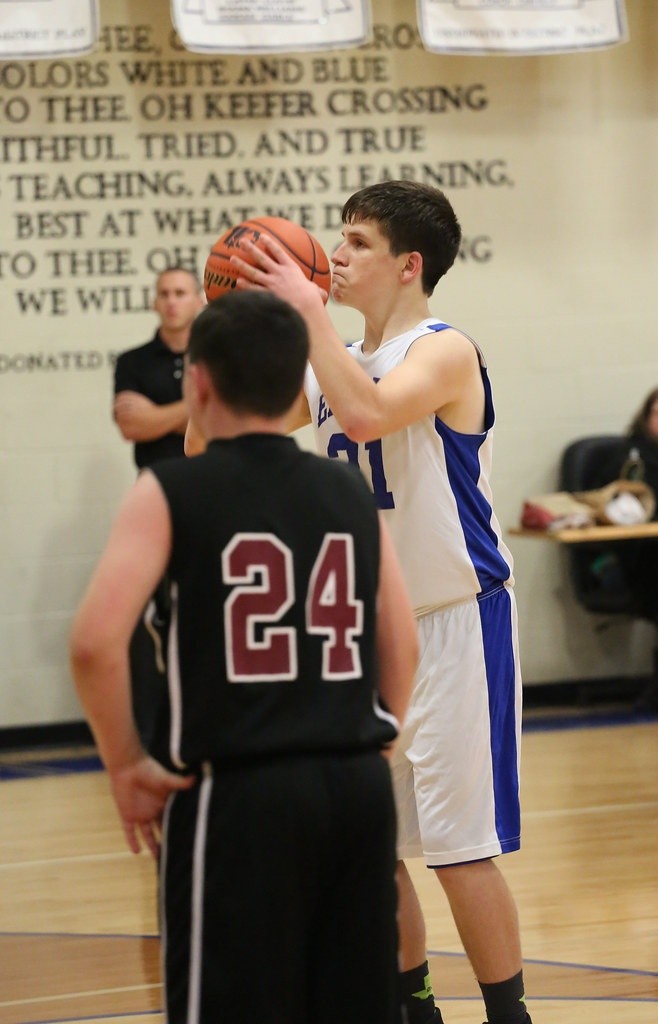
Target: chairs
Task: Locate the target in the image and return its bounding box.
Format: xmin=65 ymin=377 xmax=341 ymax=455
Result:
xmin=560 ymin=436 xmax=658 ymax=617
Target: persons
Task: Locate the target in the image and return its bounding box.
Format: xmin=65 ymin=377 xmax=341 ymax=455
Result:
xmin=113 ymin=268 xmax=203 ymax=742
xmin=184 ymin=181 xmax=533 ymax=1024
xmin=586 ymin=389 xmax=658 ymax=626
xmin=69 ymin=292 xmax=420 ymax=1024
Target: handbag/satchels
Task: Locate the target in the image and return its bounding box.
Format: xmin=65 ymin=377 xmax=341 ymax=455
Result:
xmin=519 ymin=492 xmax=594 ymax=531
xmin=588 ymin=478 xmax=655 ymax=526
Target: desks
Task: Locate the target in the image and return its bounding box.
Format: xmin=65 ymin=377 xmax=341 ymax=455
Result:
xmin=510 ymin=525 xmax=657 ymax=545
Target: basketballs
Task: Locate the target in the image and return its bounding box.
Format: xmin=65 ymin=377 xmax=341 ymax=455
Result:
xmin=203 ymin=215 xmax=334 ymax=308
